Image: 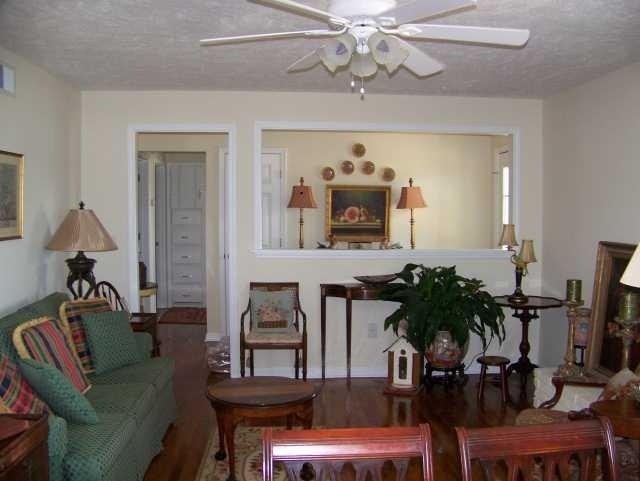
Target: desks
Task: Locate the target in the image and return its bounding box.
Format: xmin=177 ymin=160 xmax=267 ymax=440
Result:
xmin=491 ymin=295 xmax=563 ymax=401
xmin=322 ymin=282 xmax=462 ymax=388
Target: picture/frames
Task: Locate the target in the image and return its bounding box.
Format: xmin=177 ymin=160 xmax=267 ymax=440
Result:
xmin=584 ymin=241 xmax=640 ymax=395
xmin=325 ymin=184 xmax=392 ymax=242
xmin=0 ymin=150 xmax=24 ymax=240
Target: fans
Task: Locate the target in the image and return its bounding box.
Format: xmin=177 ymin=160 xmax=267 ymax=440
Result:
xmin=199 ymin=0 xmax=530 ymax=83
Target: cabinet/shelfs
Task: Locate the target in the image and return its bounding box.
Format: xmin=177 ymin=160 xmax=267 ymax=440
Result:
xmin=159 ymin=160 xmax=205 ymax=307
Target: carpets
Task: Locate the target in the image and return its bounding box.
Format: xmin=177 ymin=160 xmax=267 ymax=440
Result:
xmin=160 ymin=307 xmax=206 ymax=325
xmin=194 ymin=426 xmax=329 ymax=481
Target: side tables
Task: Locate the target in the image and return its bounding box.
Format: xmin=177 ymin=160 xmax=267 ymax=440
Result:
xmin=0 ymin=411 xmax=50 ymax=481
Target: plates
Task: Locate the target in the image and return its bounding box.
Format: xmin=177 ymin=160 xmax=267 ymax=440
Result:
xmin=353 ymin=274 xmax=398 ymax=284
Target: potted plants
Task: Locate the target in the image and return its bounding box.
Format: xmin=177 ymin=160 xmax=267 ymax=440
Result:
xmin=383 ymin=264 xmax=506 ymax=367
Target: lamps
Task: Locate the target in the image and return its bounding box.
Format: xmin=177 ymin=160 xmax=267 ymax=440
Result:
xmin=511 ymin=240 xmax=537 ymax=303
xmin=287 ymin=177 xmax=316 ymax=247
xmin=318 ymin=29 xmax=411 ymax=98
xmin=499 ymin=224 xmax=517 ymax=249
xmin=396 ymin=178 xmax=427 ymax=248
xmin=45 ymin=202 xmax=118 ymax=300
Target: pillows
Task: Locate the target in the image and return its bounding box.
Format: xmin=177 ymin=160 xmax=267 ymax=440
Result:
xmin=61 ymin=297 xmax=127 ymax=374
xmin=19 ymin=357 xmax=100 ymax=424
xmin=81 ymin=310 xmax=141 ymax=375
xmin=0 ymin=353 xmax=45 ymax=416
xmin=12 ymin=316 xmax=91 ymax=396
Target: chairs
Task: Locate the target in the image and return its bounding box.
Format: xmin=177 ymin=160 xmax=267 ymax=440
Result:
xmin=261 ymin=421 xmax=433 ymax=481
xmin=455 ymin=416 xmax=618 ymax=481
xmin=95 ymin=280 xmax=158 ymax=359
xmin=240 ymin=282 xmax=307 ymax=380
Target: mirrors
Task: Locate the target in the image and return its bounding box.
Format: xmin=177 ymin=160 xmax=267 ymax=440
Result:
xmin=253 ymin=122 xmax=520 ymax=258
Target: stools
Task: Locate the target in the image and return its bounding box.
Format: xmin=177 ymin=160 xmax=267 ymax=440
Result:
xmin=476 ymin=356 xmax=512 ymax=408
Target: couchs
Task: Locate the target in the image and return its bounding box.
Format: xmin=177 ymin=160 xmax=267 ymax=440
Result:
xmin=515 ymin=362 xmax=640 ymax=481
xmin=0 ymin=292 xmax=177 ymax=481
